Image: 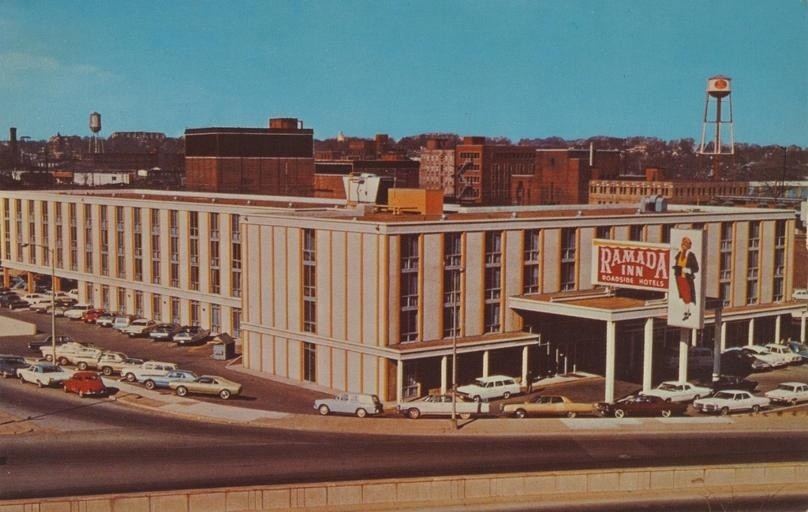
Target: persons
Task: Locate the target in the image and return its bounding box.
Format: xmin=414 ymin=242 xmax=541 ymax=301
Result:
xmin=526 ymin=371 xmax=533 ymax=393
xmin=674 ymin=236 xmax=699 ymax=320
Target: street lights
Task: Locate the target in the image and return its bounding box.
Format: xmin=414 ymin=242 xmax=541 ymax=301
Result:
xmin=21 ymin=241 xmax=55 ymax=363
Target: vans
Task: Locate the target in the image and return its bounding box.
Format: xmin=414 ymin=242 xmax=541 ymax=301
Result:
xmin=313 ymin=392 xmax=382 ymax=416
xmin=665 ymin=345 xmax=713 ymax=370
xmin=456 ymin=374 xmax=520 ymax=401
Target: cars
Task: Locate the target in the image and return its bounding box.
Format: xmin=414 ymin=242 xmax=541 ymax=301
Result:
xmin=0 ymin=273 xmax=211 ymax=344
xmin=693 ymin=389 xmax=771 ymax=416
xmin=697 ymin=375 xmax=759 ymax=390
xmin=792 ymin=289 xmax=808 ymax=300
xmin=599 ymin=394 xmax=687 ymax=418
xmin=501 ymin=394 xmax=598 ymax=417
xmin=1 ymin=333 xmax=242 ymax=400
xmin=764 ymin=381 xmax=808 ymax=406
xmin=398 ymin=394 xmax=490 ymax=419
xmin=641 ymin=381 xmax=712 ymax=403
xmin=722 ymin=340 xmax=808 ymax=372
